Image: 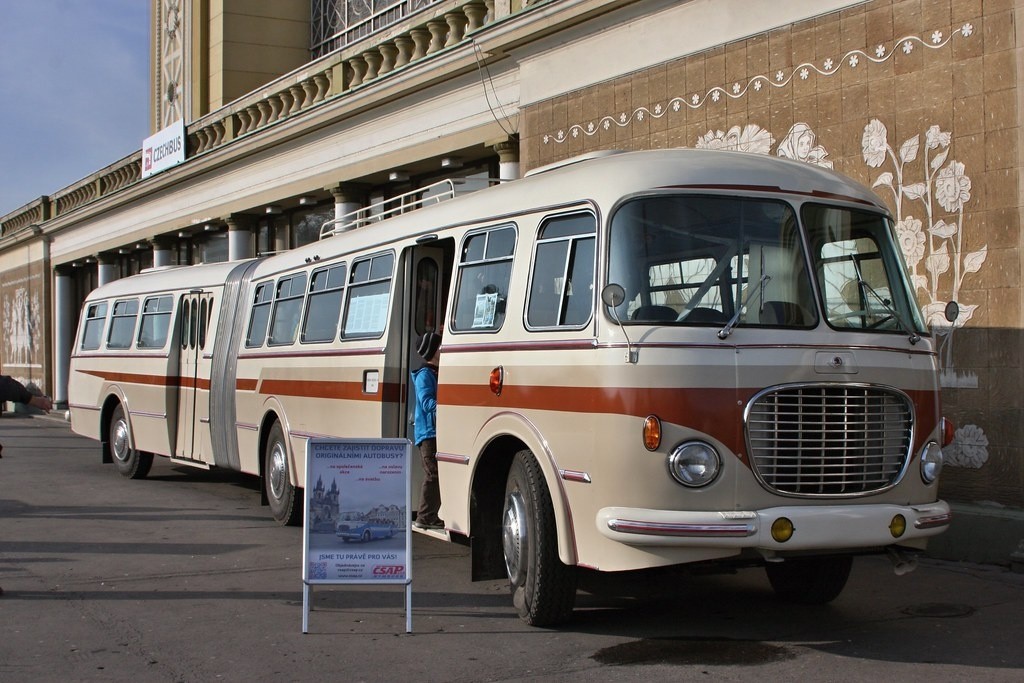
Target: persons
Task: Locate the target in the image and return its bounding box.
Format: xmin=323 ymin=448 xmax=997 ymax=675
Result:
xmin=0 ymin=375 xmax=53 ymax=415
xmin=410 ymin=332 xmax=445 ymax=530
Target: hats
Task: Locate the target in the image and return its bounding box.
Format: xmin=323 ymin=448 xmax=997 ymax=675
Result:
xmin=416 ymin=332 xmax=442 ymax=361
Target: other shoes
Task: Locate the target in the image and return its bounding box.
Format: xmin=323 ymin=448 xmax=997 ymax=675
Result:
xmin=415 ymin=517 xmax=444 ymax=529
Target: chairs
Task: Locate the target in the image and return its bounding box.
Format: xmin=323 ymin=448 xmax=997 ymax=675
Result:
xmin=683 ymin=307 xmax=729 ymax=323
xmin=631 ymin=306 xmax=678 ymax=322
xmin=760 ymin=300 xmax=804 ymax=326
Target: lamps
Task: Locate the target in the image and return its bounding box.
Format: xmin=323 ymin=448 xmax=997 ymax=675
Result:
xmin=204 ymin=225 xmax=218 ymax=231
xmin=178 ymin=232 xmax=192 ymax=237
xmin=265 ymin=207 xmax=281 ymax=213
xmin=119 ymin=250 xmax=131 ymax=254
xmin=86 ymin=259 xmax=97 ymax=263
xmin=442 ymin=159 xmax=463 ymax=167
xmin=136 ymin=244 xmax=148 ymax=249
xmin=389 ymin=173 xmax=409 ymax=181
xmin=300 ymin=197 xmax=317 ymax=206
xmin=72 ymin=263 xmax=83 ymax=267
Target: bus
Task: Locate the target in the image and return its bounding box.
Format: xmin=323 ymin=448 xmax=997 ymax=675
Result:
xmin=62 ymin=148 xmax=959 ymax=628
